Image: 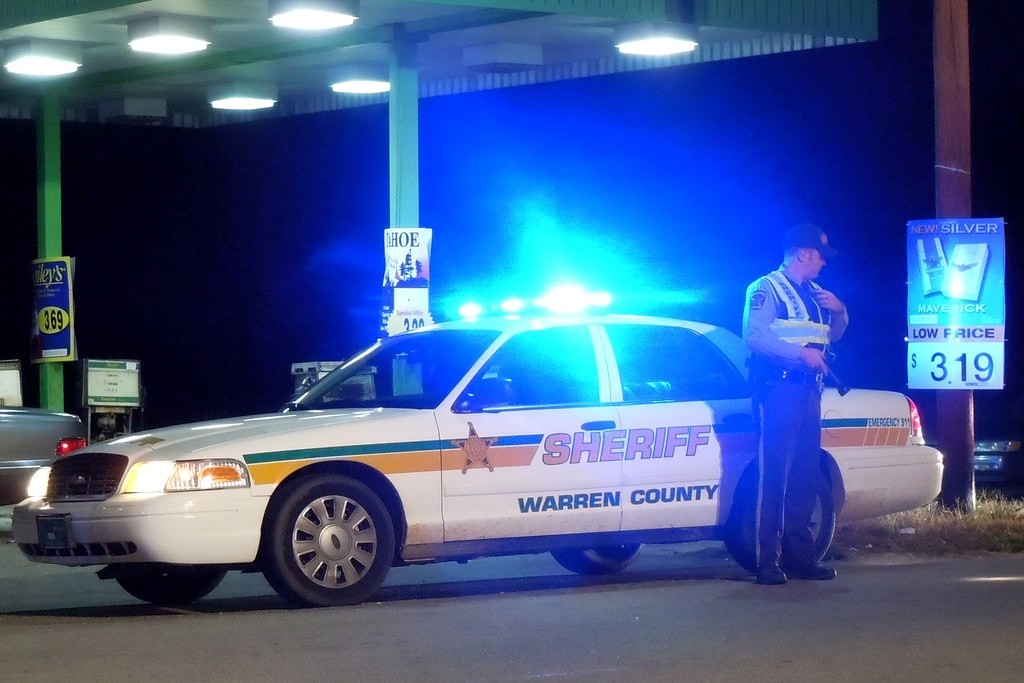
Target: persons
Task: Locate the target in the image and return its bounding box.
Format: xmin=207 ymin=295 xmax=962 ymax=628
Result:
xmin=742 ymin=224 xmax=850 ymax=584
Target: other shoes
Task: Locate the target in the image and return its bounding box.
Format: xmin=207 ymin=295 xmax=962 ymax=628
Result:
xmin=780 ymin=562 xmax=835 ymax=581
xmin=756 ymin=568 xmax=786 ymax=584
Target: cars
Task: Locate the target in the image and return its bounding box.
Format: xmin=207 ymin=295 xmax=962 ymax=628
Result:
xmin=7 ymin=282 xmax=947 ymax=609
xmin=0 ymin=403 xmax=89 ymax=508
xmin=972 ymin=421 xmax=1024 ymax=497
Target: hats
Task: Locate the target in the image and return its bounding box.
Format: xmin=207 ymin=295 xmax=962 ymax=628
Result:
xmin=785 ymin=224 xmax=838 ymax=260
